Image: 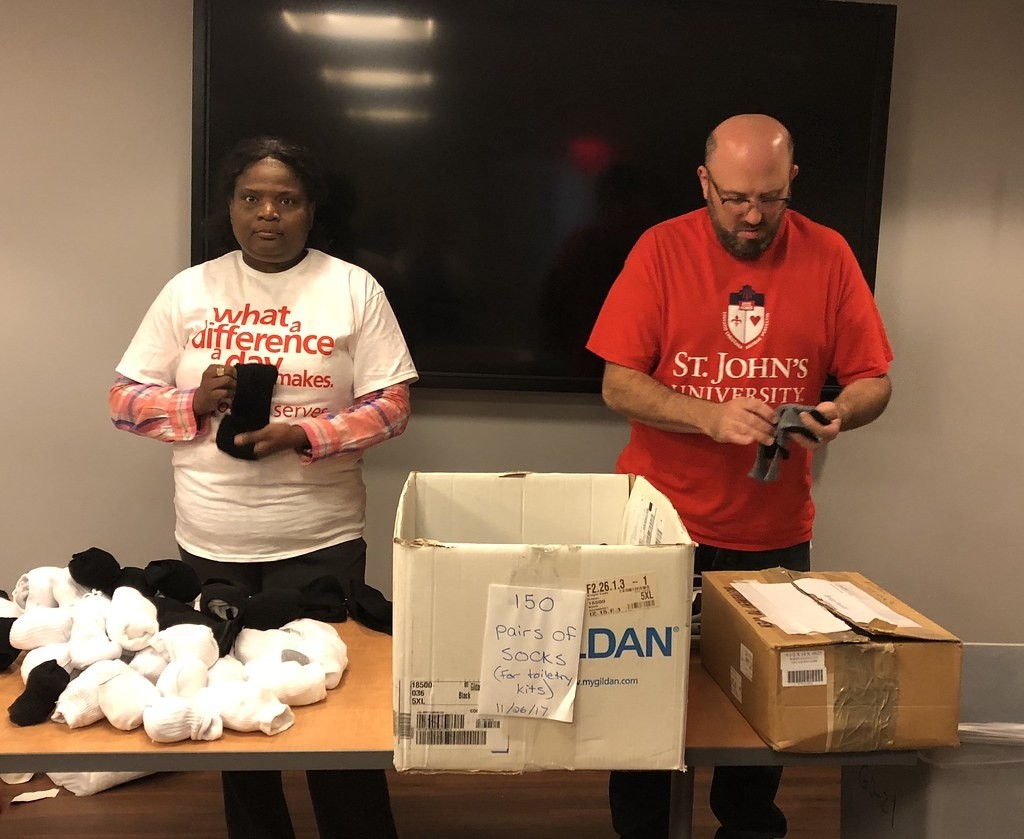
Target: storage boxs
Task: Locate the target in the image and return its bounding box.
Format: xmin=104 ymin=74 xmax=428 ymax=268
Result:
xmin=392 ymin=471 xmax=698 ymax=774
xmin=697 ymin=565 xmax=966 ymax=753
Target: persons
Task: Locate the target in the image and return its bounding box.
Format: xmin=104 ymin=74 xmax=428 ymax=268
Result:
xmin=106 ymin=133 xmax=417 ymax=839
xmin=584 ymin=113 xmax=894 ymax=839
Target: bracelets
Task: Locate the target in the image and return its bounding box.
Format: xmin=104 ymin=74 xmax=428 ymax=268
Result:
xmin=300 ymin=444 xmax=315 ymax=455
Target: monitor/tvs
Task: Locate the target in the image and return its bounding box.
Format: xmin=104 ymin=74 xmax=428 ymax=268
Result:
xmin=190 ymin=0 xmax=899 ymax=393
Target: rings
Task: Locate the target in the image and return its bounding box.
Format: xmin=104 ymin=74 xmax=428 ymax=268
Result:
xmin=215 ymin=366 xmax=225 ymax=377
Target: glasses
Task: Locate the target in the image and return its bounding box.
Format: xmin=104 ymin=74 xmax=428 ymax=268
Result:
xmin=707 ymin=171 xmax=792 ymax=214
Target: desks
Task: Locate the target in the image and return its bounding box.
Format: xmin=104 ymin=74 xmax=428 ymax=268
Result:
xmin=1 ymin=616 xmax=922 ymax=838
